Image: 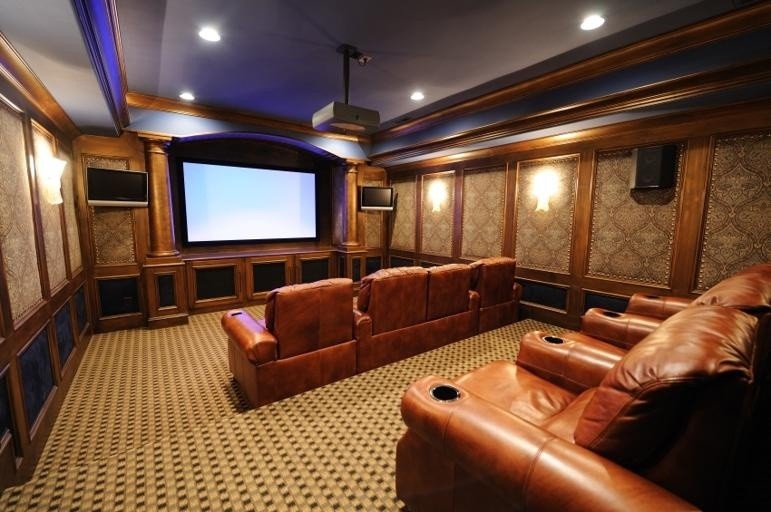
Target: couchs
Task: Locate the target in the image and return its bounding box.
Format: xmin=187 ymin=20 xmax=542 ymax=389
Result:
xmin=219 ymin=255 xmax=522 ymax=407
xmin=396 ymin=264 xmax=770 ymax=511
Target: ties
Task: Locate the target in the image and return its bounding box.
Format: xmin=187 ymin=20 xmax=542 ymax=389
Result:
xmin=313 ymin=102 xmax=380 ymax=137
xmin=360 ymin=185 xmax=394 ymax=212
xmin=86 ymin=166 xmax=149 ymax=209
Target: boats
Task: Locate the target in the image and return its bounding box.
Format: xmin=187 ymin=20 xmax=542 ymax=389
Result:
xmin=628 ymin=143 xmax=674 ymax=191
